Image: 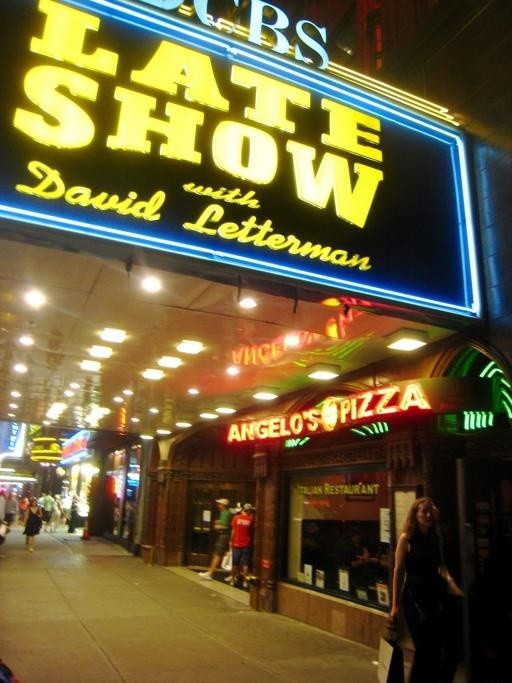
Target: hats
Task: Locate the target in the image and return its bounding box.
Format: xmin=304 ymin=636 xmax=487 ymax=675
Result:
xmin=215 ymin=498 xmax=225 ymax=505
xmin=243 ymin=503 xmax=256 ymax=511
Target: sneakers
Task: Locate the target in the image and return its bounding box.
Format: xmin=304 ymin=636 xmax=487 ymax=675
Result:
xmin=198 ymin=572 xmax=212 ymax=580
xmin=25 ymin=544 xmax=34 ymax=552
xmin=224 ymin=576 xmax=249 ymax=588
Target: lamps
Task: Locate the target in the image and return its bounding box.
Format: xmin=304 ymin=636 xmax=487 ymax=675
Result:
xmin=198 ymin=403 xmax=237 ymax=418
xmin=305 ymin=362 xmax=340 ymax=380
xmin=385 ymin=327 xmax=428 ymax=351
xmin=251 ymin=385 xmax=280 ymax=401
xmin=138 ymin=426 xmax=172 ymax=439
xmin=175 ymin=419 xmax=192 ymax=428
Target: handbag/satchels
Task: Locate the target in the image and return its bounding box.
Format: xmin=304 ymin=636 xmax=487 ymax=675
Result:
xmin=378 ymin=619 xmax=405 ymax=682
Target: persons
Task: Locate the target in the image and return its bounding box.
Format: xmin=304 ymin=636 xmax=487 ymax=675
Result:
xmin=389 ymin=497 xmax=464 ymax=683
xmin=0 ymin=489 xmax=81 ymax=552
xmin=197 ymin=497 xmax=254 ymax=588
xmin=304 ymin=523 xmax=379 ymax=588
